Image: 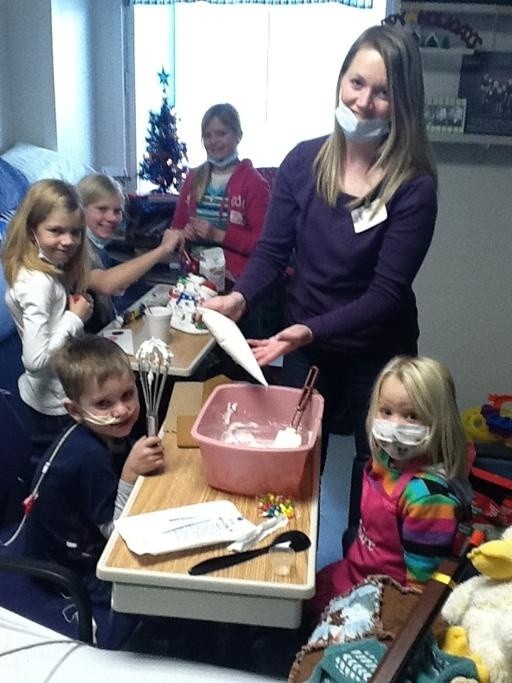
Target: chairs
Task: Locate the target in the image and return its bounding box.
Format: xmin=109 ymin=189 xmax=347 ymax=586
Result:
xmin=1 ymin=388 xmax=142 ymax=645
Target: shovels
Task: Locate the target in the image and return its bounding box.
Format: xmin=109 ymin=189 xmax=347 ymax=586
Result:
xmin=271 ymin=366 xmax=318 ymax=450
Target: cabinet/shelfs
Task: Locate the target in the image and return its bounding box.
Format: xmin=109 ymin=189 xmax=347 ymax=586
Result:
xmin=386 ymin=0 xmax=512 ymax=147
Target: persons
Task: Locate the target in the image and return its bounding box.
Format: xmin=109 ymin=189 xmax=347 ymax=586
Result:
xmin=66 ymin=174 xmax=182 ymax=329
xmin=272 ymin=352 xmax=474 ymax=677
xmin=432 ymin=105 xmax=447 ymax=125
xmin=478 ymin=71 xmax=512 ymax=114
xmin=448 ymin=105 xmax=462 ymax=125
xmin=190 ymin=24 xmax=438 ymax=558
xmin=0 ymin=178 xmax=97 ymax=474
xmin=2 ymin=334 xmax=167 ymax=649
xmin=167 ymin=102 xmax=273 ymax=337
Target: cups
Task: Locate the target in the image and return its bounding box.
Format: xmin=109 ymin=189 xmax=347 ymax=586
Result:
xmin=269 ymin=546 xmax=296 ymax=575
xmin=145 ymin=306 xmax=173 ymax=344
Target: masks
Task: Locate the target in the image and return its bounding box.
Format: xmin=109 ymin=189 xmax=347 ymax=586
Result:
xmin=86 ymin=226 xmax=111 ymax=249
xmin=372 ymin=418 xmax=431 ymax=460
xmin=335 ymin=88 xmax=391 ymax=143
xmin=207 ymin=143 xmax=238 ymax=167
